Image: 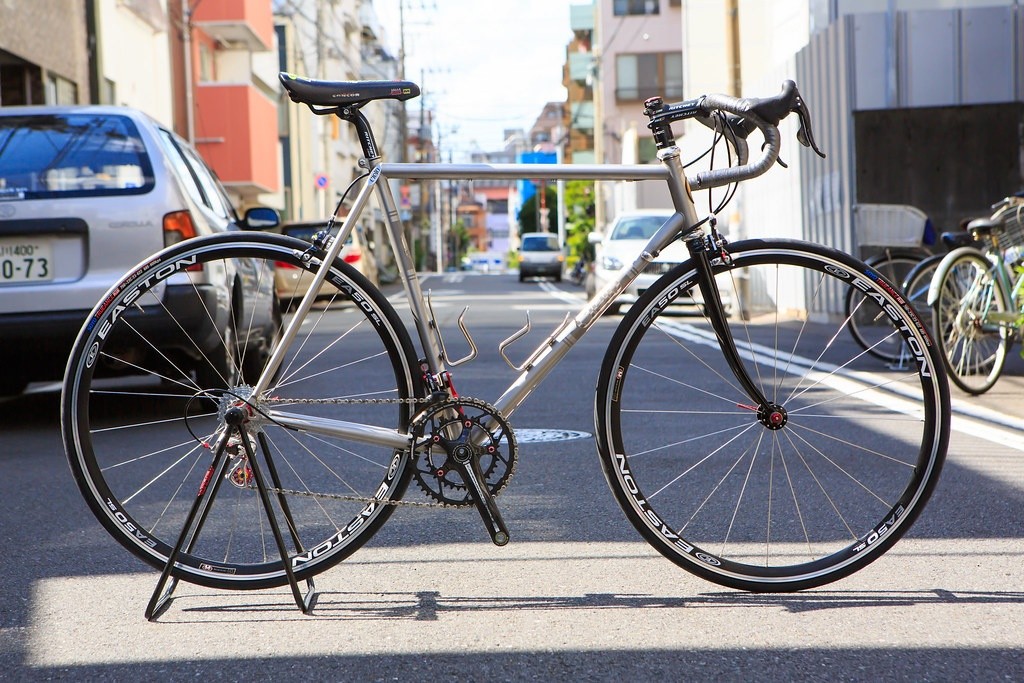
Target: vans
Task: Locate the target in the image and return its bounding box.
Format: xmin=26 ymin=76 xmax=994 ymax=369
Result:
xmin=277 ymin=218 xmax=377 ymax=301
xmin=517 ymin=231 xmax=567 ymax=283
xmin=1 ymin=105 xmax=288 ymax=413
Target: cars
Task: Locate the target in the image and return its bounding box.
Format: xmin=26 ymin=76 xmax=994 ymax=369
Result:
xmin=581 ymin=206 xmax=732 ymax=319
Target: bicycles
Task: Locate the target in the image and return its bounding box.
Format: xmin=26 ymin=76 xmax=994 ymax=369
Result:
xmin=844 ymin=193 xmax=1024 ymax=394
xmin=57 ymin=70 xmax=952 ymax=620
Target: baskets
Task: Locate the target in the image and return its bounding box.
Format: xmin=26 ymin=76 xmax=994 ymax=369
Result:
xmin=853 ymin=203 xmax=926 ymax=249
xmin=994 ymin=213 xmax=1024 ymax=247
